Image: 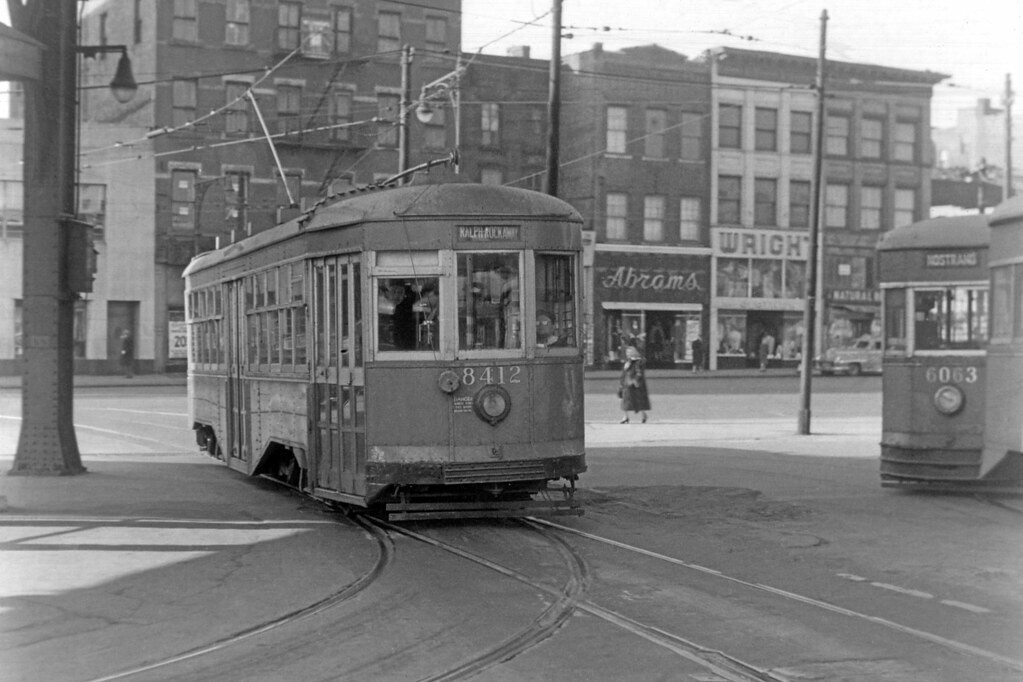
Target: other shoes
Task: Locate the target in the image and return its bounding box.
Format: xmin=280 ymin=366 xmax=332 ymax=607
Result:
xmin=620 ymin=418 xmax=629 ymax=423
xmin=641 ymin=418 xmax=645 ymax=423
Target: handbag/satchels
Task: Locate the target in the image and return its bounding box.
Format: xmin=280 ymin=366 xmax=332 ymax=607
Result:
xmin=618 ymin=387 xmax=623 ymax=399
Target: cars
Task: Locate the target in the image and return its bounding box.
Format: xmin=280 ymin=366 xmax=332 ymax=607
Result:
xmin=816 ymin=334 xmax=883 ymax=376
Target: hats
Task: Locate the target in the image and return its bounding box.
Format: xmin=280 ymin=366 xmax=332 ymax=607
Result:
xmin=536 ymin=309 xmax=556 ymax=324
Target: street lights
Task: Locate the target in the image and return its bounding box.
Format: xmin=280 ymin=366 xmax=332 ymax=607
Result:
xmin=11 ymin=43 xmax=138 ymax=475
xmin=397 ymin=43 xmax=433 ymax=184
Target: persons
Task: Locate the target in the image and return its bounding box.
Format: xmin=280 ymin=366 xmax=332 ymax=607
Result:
xmin=619 ymin=345 xmax=651 ymax=423
xmin=418 ymin=282 xmax=439 ymax=351
xmin=726 ymin=322 xmax=741 ymax=350
xmin=691 ymin=336 xmax=705 ymax=374
xmin=759 ymin=332 xmax=769 ymax=371
xmin=536 ymin=310 xmax=567 ymax=348
xmin=650 ymin=320 xmax=665 ymax=361
xmin=120 ymin=329 xmax=134 ymax=379
xmin=387 ymin=279 xmax=416 ymax=351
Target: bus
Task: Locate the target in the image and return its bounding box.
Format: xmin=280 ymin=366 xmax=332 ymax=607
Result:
xmin=181 ymin=183 xmax=590 ymax=522
xmin=872 ymin=216 xmax=1023 ymax=502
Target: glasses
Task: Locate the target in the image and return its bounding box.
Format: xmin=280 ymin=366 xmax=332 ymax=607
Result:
xmin=536 ymin=320 xmax=553 ymax=325
